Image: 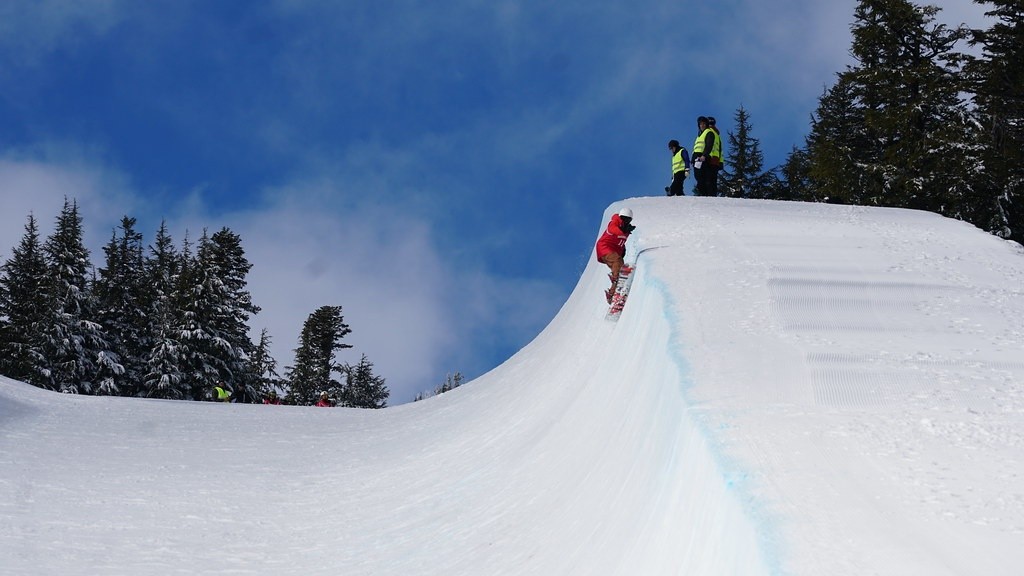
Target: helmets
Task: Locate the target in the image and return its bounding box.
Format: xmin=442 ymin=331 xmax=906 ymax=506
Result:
xmin=697 ymin=116 xmax=708 ymax=125
xmin=320 ymin=391 xmax=329 ymax=396
xmin=667 ymin=140 xmax=679 ymax=149
xmin=707 ymin=116 xmax=716 ymax=126
xmin=268 ymin=389 xmax=276 ymax=396
xmin=618 ymin=208 xmax=633 ymax=223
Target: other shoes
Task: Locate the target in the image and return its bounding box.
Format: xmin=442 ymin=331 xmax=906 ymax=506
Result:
xmin=607 ymin=272 xmax=618 ymax=283
xmin=604 ymin=289 xmax=612 ymax=303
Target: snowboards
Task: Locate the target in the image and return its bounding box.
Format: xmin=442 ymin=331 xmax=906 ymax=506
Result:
xmin=604 ymin=264 xmax=636 ymax=322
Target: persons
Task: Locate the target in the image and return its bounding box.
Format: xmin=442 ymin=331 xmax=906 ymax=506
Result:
xmin=224 ymin=382 xmax=254 ymax=403
xmin=315 ymin=391 xmax=332 ymax=407
xmin=597 ymin=208 xmax=636 ymax=304
xmin=665 ymin=139 xmax=690 ymax=196
xmin=709 ymin=116 xmax=724 ymax=196
xmin=264 ymin=390 xmax=284 ymax=405
xmin=212 ymin=380 xmax=230 ymax=402
xmin=690 ymin=116 xmax=715 ymax=196
